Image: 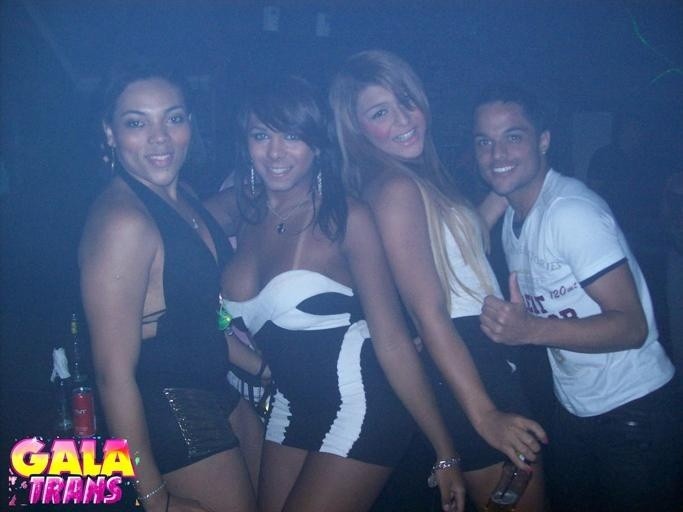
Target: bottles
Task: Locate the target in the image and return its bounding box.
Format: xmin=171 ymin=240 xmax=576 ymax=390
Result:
xmin=64 ymin=312 xmax=93 ymax=384
xmin=50 ymin=375 xmax=72 ymax=429
xmin=486 ymin=433 xmax=537 ymax=510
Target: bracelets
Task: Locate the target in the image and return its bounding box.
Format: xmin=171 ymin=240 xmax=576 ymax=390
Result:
xmin=428 ymin=456 xmax=461 ymax=488
xmin=139 ymin=481 xmax=167 ymax=501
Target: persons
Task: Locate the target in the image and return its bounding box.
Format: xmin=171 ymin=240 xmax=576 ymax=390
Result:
xmin=201 ymin=80 xmax=468 ymax=512
xmin=78 ymin=61 xmax=255 ymax=512
xmin=470 ymin=83 xmax=682 ymax=511
xmin=328 ymin=47 xmax=550 ymax=512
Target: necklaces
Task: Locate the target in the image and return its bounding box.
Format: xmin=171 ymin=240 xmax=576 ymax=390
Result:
xmin=263 ymin=194 xmax=313 ymax=235
xmin=190 ymin=219 xmax=199 ymax=232
